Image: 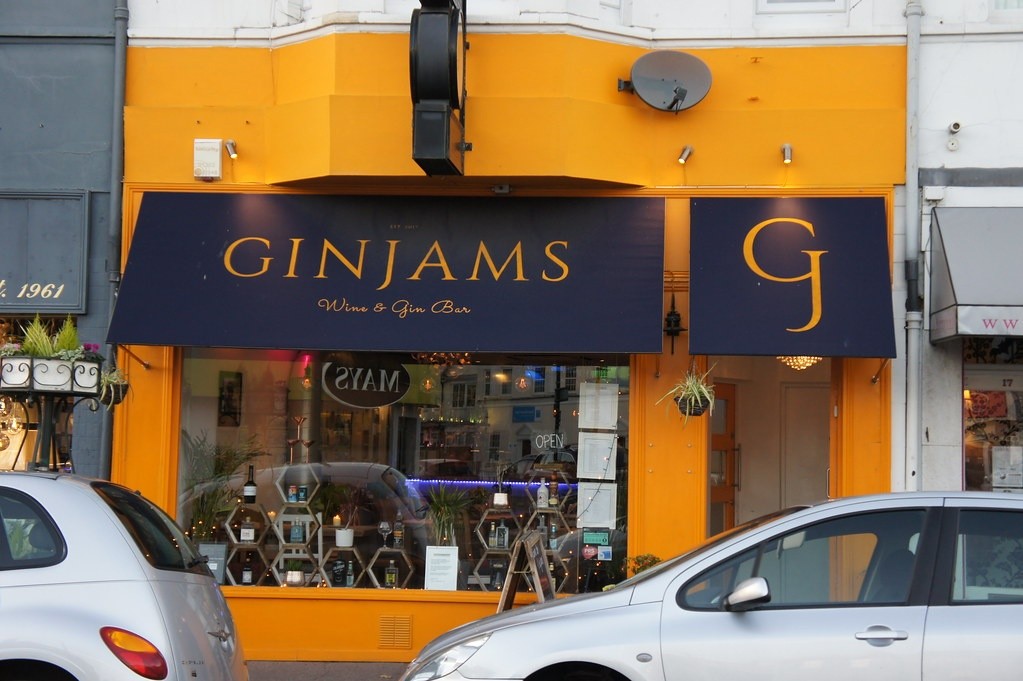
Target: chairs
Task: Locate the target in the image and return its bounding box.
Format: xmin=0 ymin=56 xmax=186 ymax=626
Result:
xmin=22 ymin=524 xmax=55 ymax=559
xmin=873 ymin=549 xmax=915 ymax=602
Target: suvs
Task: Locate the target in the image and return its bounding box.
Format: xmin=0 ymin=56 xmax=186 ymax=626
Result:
xmin=496 ymin=440 xmax=629 ymax=484
xmin=176 ymin=463 xmax=440 ymax=579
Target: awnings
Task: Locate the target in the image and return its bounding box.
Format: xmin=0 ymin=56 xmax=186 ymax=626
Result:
xmin=687 ymin=198 xmax=898 ymax=380
xmin=106 ymin=190 xmax=665 ymax=381
xmin=929 ymin=207 xmax=1023 ymax=345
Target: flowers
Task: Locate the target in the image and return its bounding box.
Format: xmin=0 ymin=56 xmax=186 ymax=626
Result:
xmin=0 ymin=342 xmax=21 ymax=355
xmin=85 ymin=343 xmax=99 ymax=353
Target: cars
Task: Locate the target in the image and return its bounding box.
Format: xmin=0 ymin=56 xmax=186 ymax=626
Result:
xmin=535 ymin=516 xmax=629 ymax=593
xmin=398 ymin=491 xmax=1023 ymax=681
xmin=0 ymin=471 xmax=250 ymax=681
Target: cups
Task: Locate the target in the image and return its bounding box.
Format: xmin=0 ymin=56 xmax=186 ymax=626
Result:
xmin=335 ymin=529 xmax=354 ymax=547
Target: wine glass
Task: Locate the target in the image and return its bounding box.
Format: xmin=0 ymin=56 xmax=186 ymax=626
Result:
xmin=378 ymin=521 xmax=393 ymax=548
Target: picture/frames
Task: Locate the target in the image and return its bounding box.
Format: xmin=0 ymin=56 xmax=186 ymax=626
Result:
xmin=218 ymin=371 xmax=242 ymax=426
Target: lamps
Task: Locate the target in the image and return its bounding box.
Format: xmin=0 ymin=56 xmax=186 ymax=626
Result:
xmin=411 ymin=352 xmax=471 ymax=378
xmin=784 ymin=146 xmax=792 ymax=163
xmin=776 ymin=356 xmax=823 ymax=370
xmin=679 ymin=147 xmax=691 ymax=164
xmin=225 ymin=143 xmax=237 ymax=158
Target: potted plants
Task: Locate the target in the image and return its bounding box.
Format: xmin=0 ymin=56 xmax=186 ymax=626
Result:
xmin=656 ymin=358 xmax=720 ymax=428
xmin=100 ymin=364 xmax=133 ymax=412
xmin=287 ymin=559 xmax=305 ymax=586
xmin=0 ymin=310 xmax=101 ymax=396
xmin=493 ymin=461 xmax=508 ymax=509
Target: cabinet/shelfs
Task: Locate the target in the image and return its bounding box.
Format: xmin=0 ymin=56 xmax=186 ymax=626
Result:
xmin=225 ymin=461 xmax=414 ymax=589
xmin=472 ymin=463 xmax=573 ymax=594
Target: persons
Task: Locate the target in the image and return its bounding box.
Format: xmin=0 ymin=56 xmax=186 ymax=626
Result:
xmin=422 ymin=447 xmax=478 ymax=480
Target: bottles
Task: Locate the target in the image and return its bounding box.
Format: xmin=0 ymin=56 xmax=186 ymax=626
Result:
xmin=289 ymin=486 xmax=296 ymax=501
xmin=241 ymin=517 xmax=254 ymax=543
xmin=385 ymin=560 xmax=398 ymax=587
xmin=549 ymin=475 xmax=558 ymax=506
xmin=394 ymin=519 xmax=404 ymax=548
xmin=291 ymin=519 xmax=303 ymax=541
xmin=489 ymin=521 xmax=496 ymax=548
xmin=333 ymin=552 xmax=346 ymax=586
xmin=241 ymin=557 xmax=252 ymax=585
xmin=243 ymin=464 xmax=256 ymax=504
xmin=346 ymin=561 xmax=354 ymax=586
xmin=549 ymin=527 xmax=557 ymax=549
xmin=537 ymin=478 xmax=549 ymax=507
xmin=496 ymin=518 xmax=508 ymax=549
xmin=537 ymin=517 xmax=547 ymax=547
xmin=299 ymin=486 xmax=307 ymax=502
